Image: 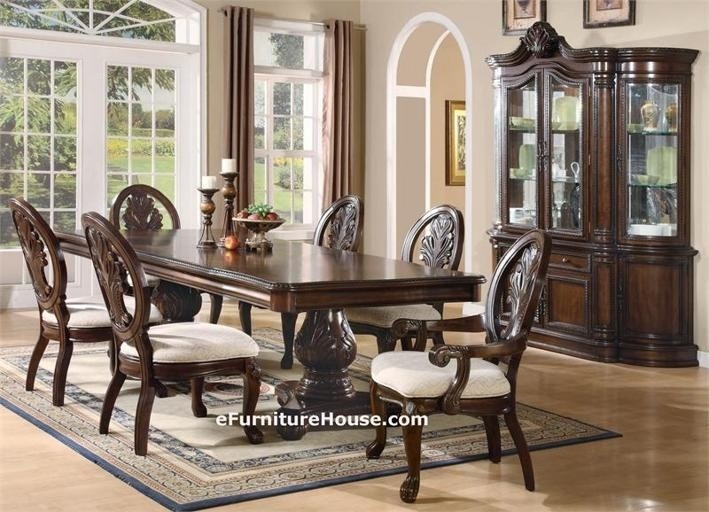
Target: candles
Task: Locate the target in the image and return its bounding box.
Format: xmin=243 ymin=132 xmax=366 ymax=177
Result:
xmin=222 ymin=157 xmax=236 ymax=172
xmin=202 ymin=175 xmax=216 ymax=189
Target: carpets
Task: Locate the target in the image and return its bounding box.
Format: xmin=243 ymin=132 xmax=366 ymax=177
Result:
xmin=0 ymin=325 xmax=623 ymax=512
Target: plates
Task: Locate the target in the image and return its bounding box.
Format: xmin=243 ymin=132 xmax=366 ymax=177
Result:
xmin=647 ymin=147 xmax=678 ymax=185
xmin=554 ymin=97 xmax=580 ymax=130
xmin=518 ymin=144 xmax=535 ymax=175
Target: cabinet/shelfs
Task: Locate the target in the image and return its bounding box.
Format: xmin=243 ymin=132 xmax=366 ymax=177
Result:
xmin=489 ymin=21 xmax=701 ymax=370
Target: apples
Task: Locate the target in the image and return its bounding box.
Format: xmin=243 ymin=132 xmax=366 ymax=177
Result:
xmin=265 ymin=212 xmax=280 ymax=221
xmin=236 ymin=210 xmax=248 ymax=217
xmin=247 ymin=215 xmax=264 ymax=220
xmin=224 ymin=236 xmax=238 ymax=249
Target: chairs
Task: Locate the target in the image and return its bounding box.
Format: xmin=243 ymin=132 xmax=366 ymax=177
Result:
xmin=9 ymin=196 xmax=163 ymax=406
xmin=80 ymin=211 xmax=264 ymax=455
xmin=366 ymin=228 xmax=552 ymax=504
xmin=346 ymin=202 xmax=464 ymax=355
xmin=110 ymin=183 xmax=224 ymax=324
xmin=234 ymin=193 xmax=364 ymax=369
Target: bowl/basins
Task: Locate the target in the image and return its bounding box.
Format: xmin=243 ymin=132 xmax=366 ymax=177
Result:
xmin=511 ymin=117 xmax=534 ymax=129
xmin=637 ymin=175 xmax=659 ymax=186
xmin=512 ymin=168 xmax=527 ymax=177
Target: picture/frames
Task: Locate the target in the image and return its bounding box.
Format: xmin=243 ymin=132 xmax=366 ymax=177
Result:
xmin=501 ymin=1 xmax=551 ymax=37
xmin=444 ymin=98 xmax=467 ymax=188
xmin=580 ymin=0 xmax=638 ymax=31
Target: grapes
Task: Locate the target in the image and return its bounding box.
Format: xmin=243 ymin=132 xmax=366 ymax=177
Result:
xmin=247 ymin=203 xmax=273 ymax=218
